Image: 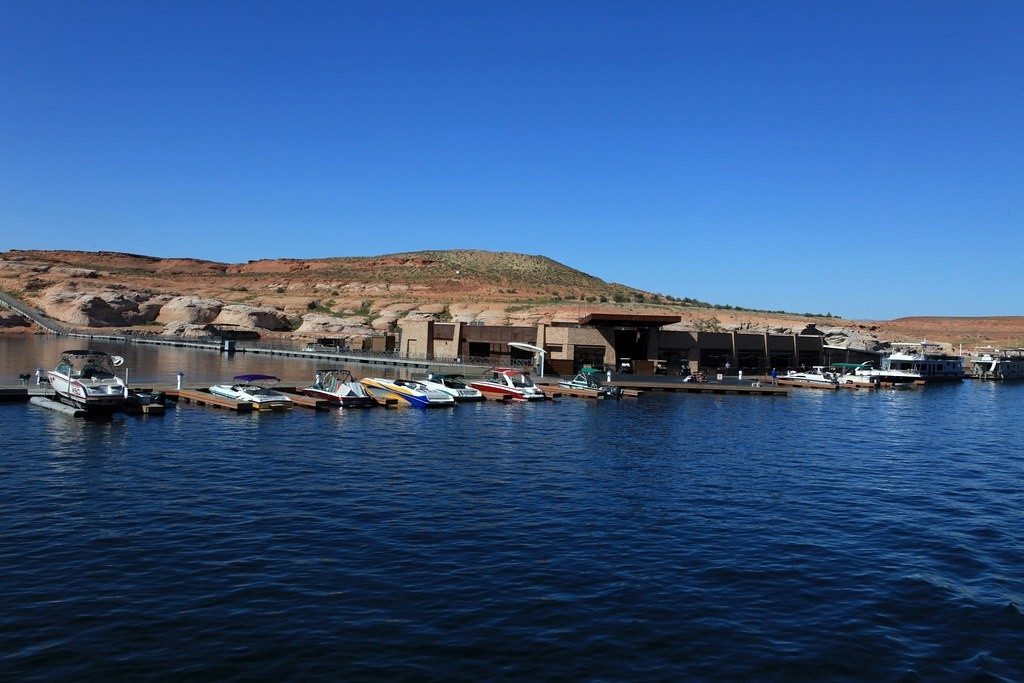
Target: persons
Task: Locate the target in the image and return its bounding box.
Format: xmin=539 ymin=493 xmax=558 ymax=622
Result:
xmin=725 ymin=361 xmax=730 ymax=368
xmin=801 ymin=364 xmax=805 ymax=370
xmin=771 ymin=368 xmax=777 ymax=385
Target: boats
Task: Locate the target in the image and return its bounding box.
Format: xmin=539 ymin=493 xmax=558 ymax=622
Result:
xmin=210 ymin=374 xmax=294 ymax=412
xmin=359 ymin=377 xmax=457 ymax=407
xmin=853 ymin=338 xmax=965 ymax=382
xmin=556 ymin=368 xmax=624 ymax=398
xmin=825 ymin=363 xmax=878 ymax=384
xmin=301 ymin=368 xmax=371 ymax=405
xmin=777 ymin=366 xmax=838 ymax=385
xmin=683 ymin=372 xmax=708 ymax=383
xmin=970 ymin=348 xmax=1024 ymax=381
xmin=471 ymin=369 xmax=546 ymax=401
xmin=303 ymin=343 xmax=339 ymax=352
xmin=47 ymin=349 xmax=131 ymax=408
xmin=416 ymin=373 xmax=483 ymax=400
